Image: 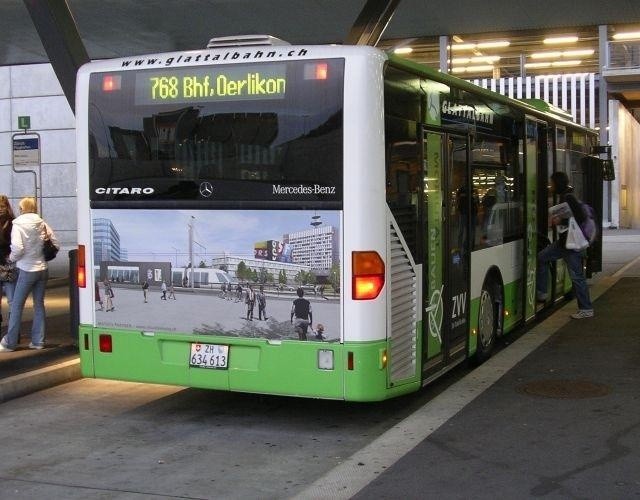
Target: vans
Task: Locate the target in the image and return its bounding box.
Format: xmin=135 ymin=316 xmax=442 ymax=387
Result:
xmin=480 ymin=201 xmax=522 ymax=243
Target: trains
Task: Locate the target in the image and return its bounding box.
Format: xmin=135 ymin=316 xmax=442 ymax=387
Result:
xmin=95 ymin=264 xmax=243 ymax=294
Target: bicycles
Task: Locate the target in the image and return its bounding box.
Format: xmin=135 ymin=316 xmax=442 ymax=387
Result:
xmin=218 ymin=289 xmax=244 ymax=304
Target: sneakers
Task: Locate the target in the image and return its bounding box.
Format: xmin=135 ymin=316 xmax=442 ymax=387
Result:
xmin=536 ymin=290 xmax=547 ymax=302
xmin=1 ymin=344 xmax=18 ymax=352
xmin=569 ymin=309 xmax=594 ymax=319
xmin=29 ymin=342 xmax=45 ymax=351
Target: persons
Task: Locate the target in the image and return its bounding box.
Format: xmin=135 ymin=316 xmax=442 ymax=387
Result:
xmin=0 ymin=197 xmax=60 ymax=352
xmin=456 ymin=172 xmax=508 ymax=261
xmin=95 ymin=276 xmax=329 ymax=342
xmin=0 ymin=196 xmax=20 ymax=345
xmin=537 ymin=171 xmax=595 ymax=319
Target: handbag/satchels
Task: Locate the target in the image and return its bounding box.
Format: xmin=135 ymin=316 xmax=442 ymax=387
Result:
xmin=566 ymin=201 xmax=596 ymax=251
xmin=42 ymin=239 xmax=60 ymax=260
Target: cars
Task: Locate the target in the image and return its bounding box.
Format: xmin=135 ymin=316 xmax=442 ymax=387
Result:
xmin=298 ymin=284 xmax=320 ymax=289
xmin=276 ymin=282 xmax=295 ymax=291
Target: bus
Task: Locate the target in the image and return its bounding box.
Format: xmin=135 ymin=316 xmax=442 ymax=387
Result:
xmin=75 ymin=31 xmax=616 ymax=413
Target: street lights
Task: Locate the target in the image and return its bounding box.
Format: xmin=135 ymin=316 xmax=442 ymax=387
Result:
xmin=333 ymin=271 xmax=337 ymax=297
xmin=300 ymin=114 xmax=309 ymax=136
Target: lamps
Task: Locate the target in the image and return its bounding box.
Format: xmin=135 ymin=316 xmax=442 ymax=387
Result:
xmin=388 ymin=29 xmax=640 ymax=77
